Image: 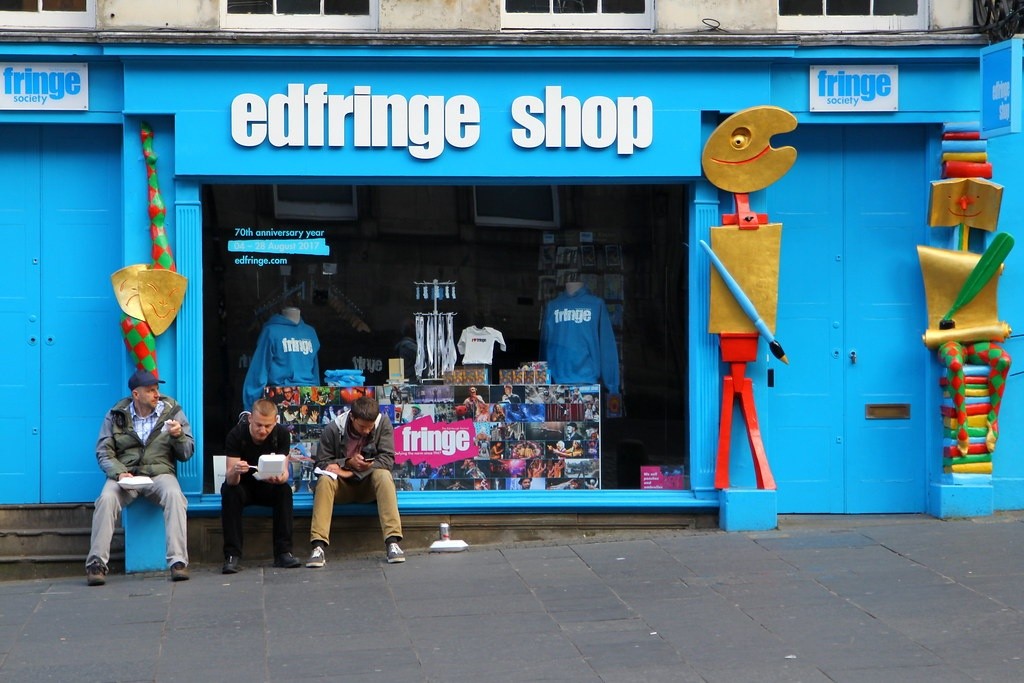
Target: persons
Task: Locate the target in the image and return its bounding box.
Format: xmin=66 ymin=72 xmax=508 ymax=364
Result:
xmin=389 ymin=386 xmax=600 ymax=490
xmin=84 ymin=370 xmax=196 ymax=587
xmin=266 ymin=387 xmax=349 ymax=481
xmin=539 ymin=281 xmax=622 ymax=396
xmin=305 ymin=397 xmax=406 ymax=567
xmin=219 ymin=398 xmax=303 ymax=574
xmin=240 ymin=307 xmax=321 ymax=413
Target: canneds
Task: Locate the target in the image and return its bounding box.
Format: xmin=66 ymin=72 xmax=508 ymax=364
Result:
xmin=439 ymin=523 xmax=450 ymax=541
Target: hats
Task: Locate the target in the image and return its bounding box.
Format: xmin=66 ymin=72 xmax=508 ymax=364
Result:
xmin=567 ymin=423 xmax=577 ymax=428
xmin=411 ymin=405 xmax=421 ymax=410
xmin=128 ymin=369 xmax=165 ymax=389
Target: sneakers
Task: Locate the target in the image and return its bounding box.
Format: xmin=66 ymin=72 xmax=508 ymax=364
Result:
xmin=387 ymin=543 xmax=405 ymax=563
xmin=222 ymin=557 xmax=240 ymax=573
xmin=172 ymin=563 xmax=189 ymax=581
xmin=273 ymin=554 xmax=301 ymax=568
xmin=87 ymin=562 xmax=104 ymax=586
xmin=306 ymin=548 xmax=325 ymax=567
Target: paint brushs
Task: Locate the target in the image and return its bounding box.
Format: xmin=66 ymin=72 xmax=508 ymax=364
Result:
xmin=699 ymin=238 xmax=790 ymax=366
